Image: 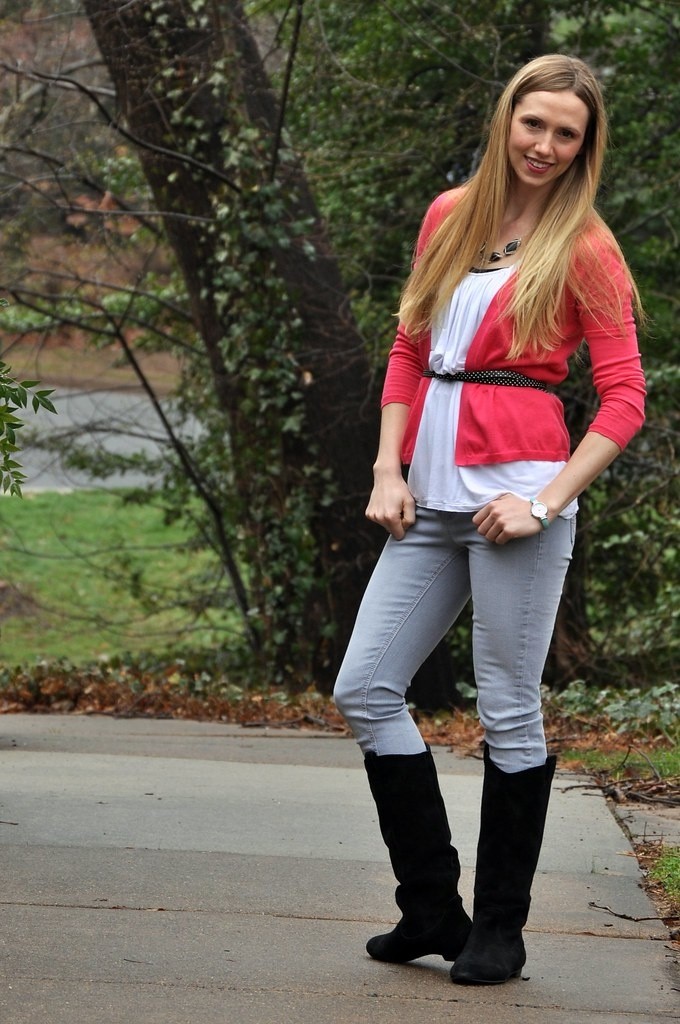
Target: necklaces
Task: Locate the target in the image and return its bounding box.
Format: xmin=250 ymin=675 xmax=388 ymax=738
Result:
xmin=477 ymin=230 xmax=523 ymax=265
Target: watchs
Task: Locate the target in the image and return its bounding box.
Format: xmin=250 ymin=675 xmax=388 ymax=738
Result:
xmin=529 ymin=495 xmax=552 ymax=535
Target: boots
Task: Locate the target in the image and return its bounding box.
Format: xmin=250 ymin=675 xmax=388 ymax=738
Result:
xmin=364 ymin=741 xmax=473 ymax=962
xmin=450 ymin=749 xmax=562 ymax=983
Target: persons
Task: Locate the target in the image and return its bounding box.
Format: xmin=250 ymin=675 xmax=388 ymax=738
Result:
xmin=329 ymin=52 xmax=649 ymax=985
